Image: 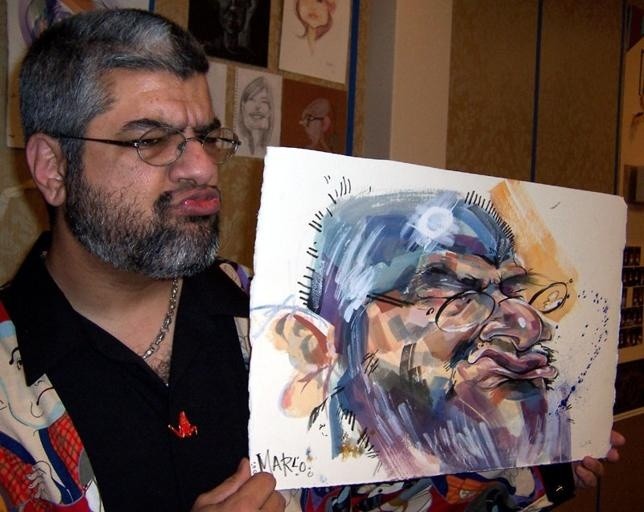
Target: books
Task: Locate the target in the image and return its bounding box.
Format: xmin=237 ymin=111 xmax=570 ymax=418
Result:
xmin=613 ymin=245 xmax=644 ymax=350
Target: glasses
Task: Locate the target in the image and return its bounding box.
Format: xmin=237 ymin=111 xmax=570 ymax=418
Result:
xmin=63 ymin=127 xmax=240 ymax=165
xmin=367 ymin=275 xmax=568 ymax=334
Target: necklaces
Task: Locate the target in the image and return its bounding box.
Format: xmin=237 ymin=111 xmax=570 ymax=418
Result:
xmin=139 ymin=273 xmax=180 ymax=362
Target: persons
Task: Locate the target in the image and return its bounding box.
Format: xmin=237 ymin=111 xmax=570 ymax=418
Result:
xmin=300 ymin=97 xmax=333 ymax=153
xmin=0 ymin=7 xmax=625 ymax=512
xmin=293 ymin=0 xmax=336 ymax=47
xmin=270 ymin=189 xmax=573 ymax=478
xmin=236 ymin=75 xmax=275 ymax=156
